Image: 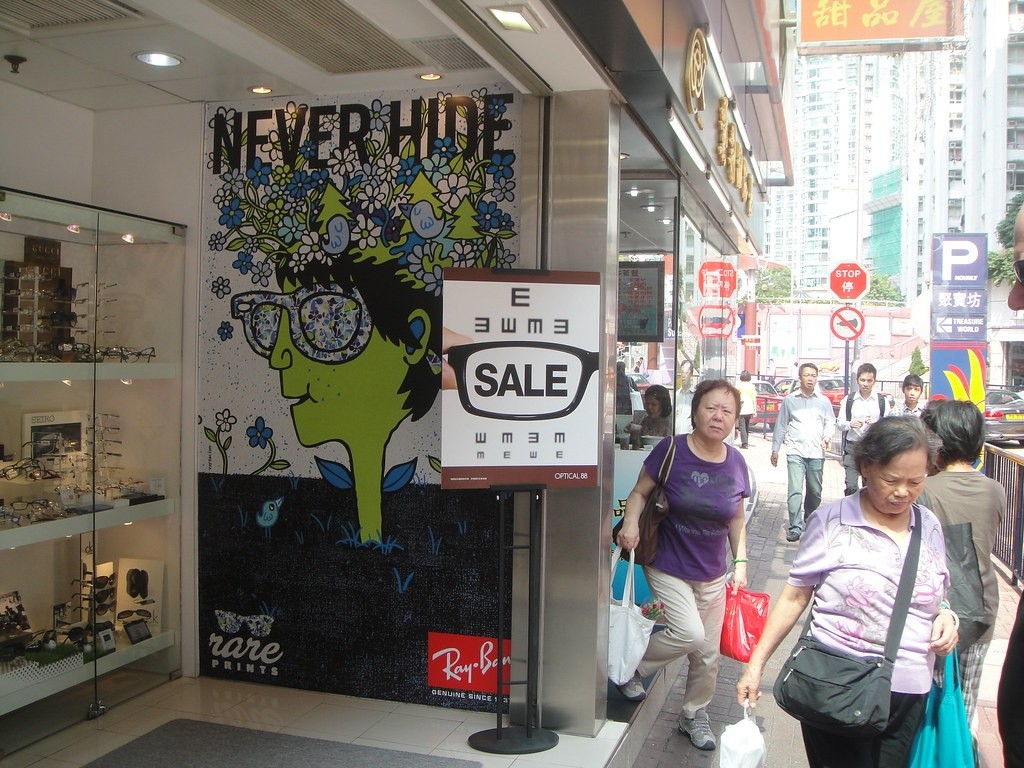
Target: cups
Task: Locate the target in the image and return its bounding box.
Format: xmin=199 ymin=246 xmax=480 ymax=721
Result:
xmin=620 ymin=433 xmax=630 ymax=449
xmin=631 ymin=424 xmax=642 ymax=450
xmin=643 ymin=445 xmax=653 ymax=449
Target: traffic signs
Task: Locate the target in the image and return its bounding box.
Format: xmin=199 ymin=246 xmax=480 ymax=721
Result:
xmin=931 ymin=313 xmax=987 ymax=342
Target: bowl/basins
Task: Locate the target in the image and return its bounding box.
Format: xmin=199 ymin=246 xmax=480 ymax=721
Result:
xmin=641 ymin=436 xmax=665 ymax=448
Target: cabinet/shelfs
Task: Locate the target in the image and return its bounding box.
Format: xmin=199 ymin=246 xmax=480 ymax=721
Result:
xmin=0 ymin=186 xmax=189 ymax=764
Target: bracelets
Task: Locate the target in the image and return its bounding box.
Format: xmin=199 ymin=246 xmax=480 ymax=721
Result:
xmin=944 ymin=609 xmax=959 ymax=630
xmin=732 ymin=559 xmax=749 ymax=563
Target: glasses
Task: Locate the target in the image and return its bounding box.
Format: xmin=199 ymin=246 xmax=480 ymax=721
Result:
xmin=0 ymin=573 xmax=152 ymax=661
xmin=0 ymin=266 xmax=155 ymax=364
xmin=801 ymin=374 xmax=816 ymax=379
xmin=0 ymin=414 xmax=124 ymax=527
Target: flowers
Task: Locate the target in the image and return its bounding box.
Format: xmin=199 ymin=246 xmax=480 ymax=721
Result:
xmin=639 ymin=597 xmax=665 ymax=621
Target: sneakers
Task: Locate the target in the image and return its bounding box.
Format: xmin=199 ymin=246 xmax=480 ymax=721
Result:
xmin=617 ymin=672 xmax=648 ymax=701
xmin=677 ymin=710 xmax=717 ymax=750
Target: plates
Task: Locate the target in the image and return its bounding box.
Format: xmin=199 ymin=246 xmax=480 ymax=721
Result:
xmin=639 ymin=448 xmax=654 ymax=451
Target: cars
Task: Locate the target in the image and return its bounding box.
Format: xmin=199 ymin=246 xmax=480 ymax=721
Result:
xmin=626 ymin=372 xmax=653 ymax=406
xmin=983 ymin=387 xmax=1024 ymax=448
xmin=729 ymin=373 xmax=896 ymax=431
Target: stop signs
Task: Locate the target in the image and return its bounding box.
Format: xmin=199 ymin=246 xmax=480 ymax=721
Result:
xmin=828 ymin=263 xmax=869 ymax=302
xmin=699 ymin=261 xmax=738 ymax=298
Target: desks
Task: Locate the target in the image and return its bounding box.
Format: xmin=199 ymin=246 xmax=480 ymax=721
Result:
xmin=611 ymin=445 xmax=654 ymax=552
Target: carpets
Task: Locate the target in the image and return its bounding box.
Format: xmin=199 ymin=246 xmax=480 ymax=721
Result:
xmin=80 ymin=718 xmax=482 ymax=768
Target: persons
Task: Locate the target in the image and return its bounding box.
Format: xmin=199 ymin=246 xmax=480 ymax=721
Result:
xmin=888 ymin=374 xmax=926 ymax=416
xmin=736 ymin=416 xmax=959 ymax=768
xmin=634 ymin=357 xmax=647 ymax=374
xmin=771 ymin=363 xmax=835 ymax=542
xmin=914 ymin=400 xmax=1005 ymax=768
xmin=616 ymin=361 xmax=636 ymax=415
xmin=624 ymin=385 xmax=671 ymax=447
xmin=616 ymin=380 xmax=751 ymax=750
xmin=837 ymin=362 xmax=891 ymax=497
xmin=676 ymin=360 xmax=695 ymax=434
xmin=734 ymin=370 xmax=757 ymax=449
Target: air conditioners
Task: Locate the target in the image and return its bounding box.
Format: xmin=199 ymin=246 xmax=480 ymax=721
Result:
xmin=950 ymin=142 xmax=956 ymax=147
xmin=949 ymin=171 xmax=956 ymax=176
xmin=1007 ymin=120 xmax=1018 ymax=127
xmin=1007 ymin=206 xmax=1014 ymax=211
xmin=949 ymin=200 xmax=955 ymax=205
xmin=1009 ymin=34 xmax=1018 ymax=43
xmin=949 ymin=229 xmax=955 ymax=233
xmin=1007 ymin=162 xmax=1016 ymax=170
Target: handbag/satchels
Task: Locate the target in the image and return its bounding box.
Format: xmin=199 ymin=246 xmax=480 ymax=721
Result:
xmin=941 ymin=522 xmax=992 ymax=656
xmin=907 ymin=644 xmax=975 ymax=768
xmin=773 ymin=636 xmax=890 ymax=736
xmin=609 ymin=542 xmax=657 ymax=686
xmin=720 ymin=698 xmax=767 ymax=768
xmin=720 ymin=583 xmax=770 ymax=663
xmin=612 ymin=436 xmax=677 ymax=565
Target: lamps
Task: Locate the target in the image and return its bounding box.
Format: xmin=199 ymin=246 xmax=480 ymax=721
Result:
xmin=121 ymin=378 xmax=133 ymax=385
xmin=701 ymin=23 xmax=762 ymax=186
xmin=61 ymin=380 xmax=72 ymax=386
xmin=663 ymin=104 xmax=758 ymax=257
xmin=121 ymin=234 xmax=134 ymax=244
xmin=484 ymin=2 xmax=549 ymax=34
xmin=66 ymin=224 xmax=80 ymax=234
xmin=0 ymin=212 xmax=11 ymax=221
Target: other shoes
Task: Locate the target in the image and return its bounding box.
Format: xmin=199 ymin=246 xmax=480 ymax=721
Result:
xmin=787 ymin=532 xmax=799 ymax=541
xmin=742 ymin=443 xmax=747 ymax=449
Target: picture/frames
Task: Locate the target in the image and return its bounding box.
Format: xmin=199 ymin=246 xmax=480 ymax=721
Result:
xmin=123 ymin=618 xmax=152 ymax=645
